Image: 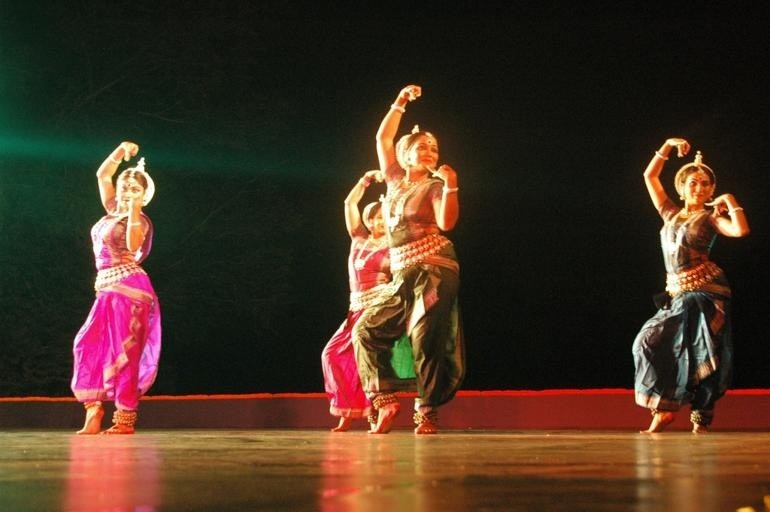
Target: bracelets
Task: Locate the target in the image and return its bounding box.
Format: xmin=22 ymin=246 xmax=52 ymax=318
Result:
xmin=126 ymin=221 xmax=142 ymax=227
xmin=654 ymin=151 xmax=670 ymax=163
xmin=107 ymin=154 xmax=123 ymax=164
xmin=356 ymin=177 xmax=371 ymax=190
xmin=726 ymin=207 xmax=745 ymax=216
xmin=442 ymin=185 xmax=460 ymax=194
xmin=390 ymin=103 xmax=406 ymax=115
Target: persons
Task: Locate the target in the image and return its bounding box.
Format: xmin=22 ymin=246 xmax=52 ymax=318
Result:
xmin=321 ymin=432 xmax=464 ymax=511
xmin=64 ymin=435 xmax=164 ymax=511
xmin=631 ymin=137 xmax=749 ymax=436
xmin=319 ymin=169 xmax=395 ymax=432
xmin=634 ymin=436 xmax=720 ymax=511
xmin=350 ymin=84 xmax=468 ymax=436
xmin=70 ymin=142 xmax=163 ymax=436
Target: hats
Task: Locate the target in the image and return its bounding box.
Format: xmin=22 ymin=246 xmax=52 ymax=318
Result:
xmin=674 ymin=161 xmax=716 ymax=201
xmin=361 ymin=200 xmax=381 ymax=232
xmin=115 ymin=167 xmax=155 ymax=207
xmin=394 ymin=134 xmax=414 ymax=170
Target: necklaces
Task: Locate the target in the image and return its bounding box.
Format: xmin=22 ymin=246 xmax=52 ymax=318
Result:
xmin=385 ymin=177 xmax=431 ymax=234
xmin=354 ymin=236 xmax=389 ymax=272
xmin=666 ymin=208 xmax=704 ymax=259
